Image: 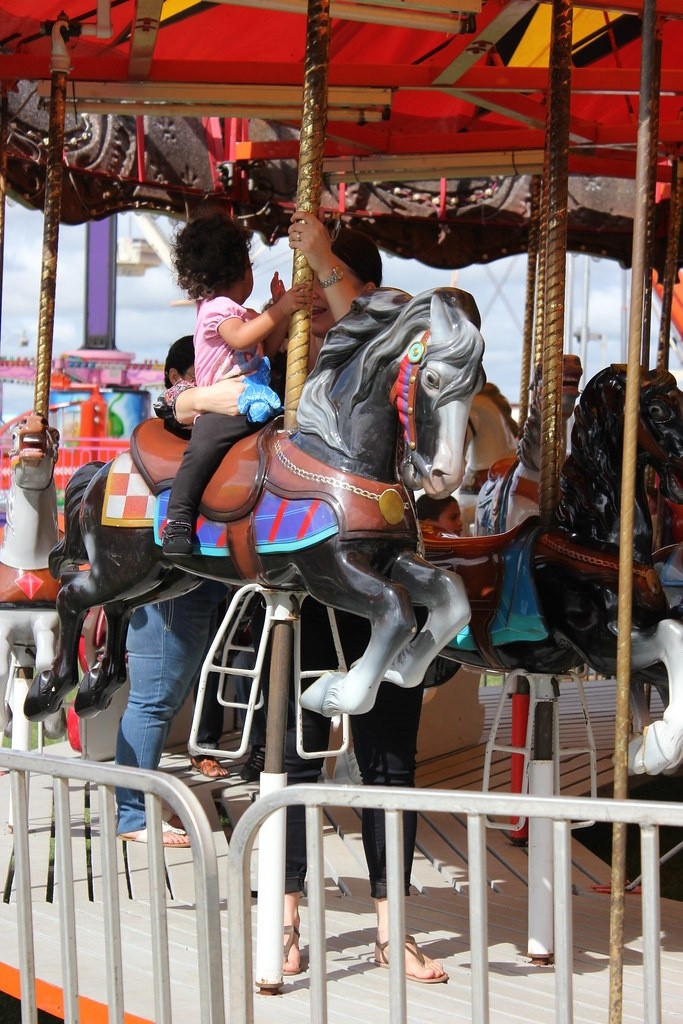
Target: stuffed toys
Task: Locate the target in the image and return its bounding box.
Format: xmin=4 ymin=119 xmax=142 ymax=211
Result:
xmin=238 ymin=355 xmax=281 ymax=422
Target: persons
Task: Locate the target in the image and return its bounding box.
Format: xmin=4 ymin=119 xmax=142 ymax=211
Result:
xmin=162 ymin=211 xmax=314 ymax=556
xmin=113 ymin=336 xmax=265 ymax=848
xmin=167 ymin=210 xmax=448 ymax=983
xmin=417 ymin=494 xmax=464 ymax=537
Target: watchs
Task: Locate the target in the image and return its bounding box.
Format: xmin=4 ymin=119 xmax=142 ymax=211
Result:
xmin=318 ymin=267 xmax=344 ymax=288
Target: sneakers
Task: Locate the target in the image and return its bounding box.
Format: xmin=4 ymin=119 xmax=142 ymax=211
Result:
xmin=239 ymin=749 xmax=265 ymax=781
xmin=161 ymin=520 xmax=194 ymax=556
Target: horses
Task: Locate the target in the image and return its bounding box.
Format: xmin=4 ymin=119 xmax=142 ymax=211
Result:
xmin=22 ymin=287 xmax=486 ymax=721
xmin=0 ymin=412 xmax=68 ymax=739
xmin=472 ymin=355 xmax=584 ymax=537
xmin=452 ymin=383 xmax=519 ymax=536
xmin=420 ymin=364 xmax=682 ymax=776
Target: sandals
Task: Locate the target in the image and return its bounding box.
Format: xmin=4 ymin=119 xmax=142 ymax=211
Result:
xmin=283 ymin=925 xmax=303 ymax=976
xmin=188 ymin=755 xmax=230 ymax=779
xmin=373 ymin=934 xmax=448 ymax=983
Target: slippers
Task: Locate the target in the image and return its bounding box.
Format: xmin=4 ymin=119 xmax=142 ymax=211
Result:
xmin=118 ymin=807 xmax=191 ymax=848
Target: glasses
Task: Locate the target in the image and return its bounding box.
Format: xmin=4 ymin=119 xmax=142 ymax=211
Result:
xmin=177 ymin=365 xmax=195 ymax=382
